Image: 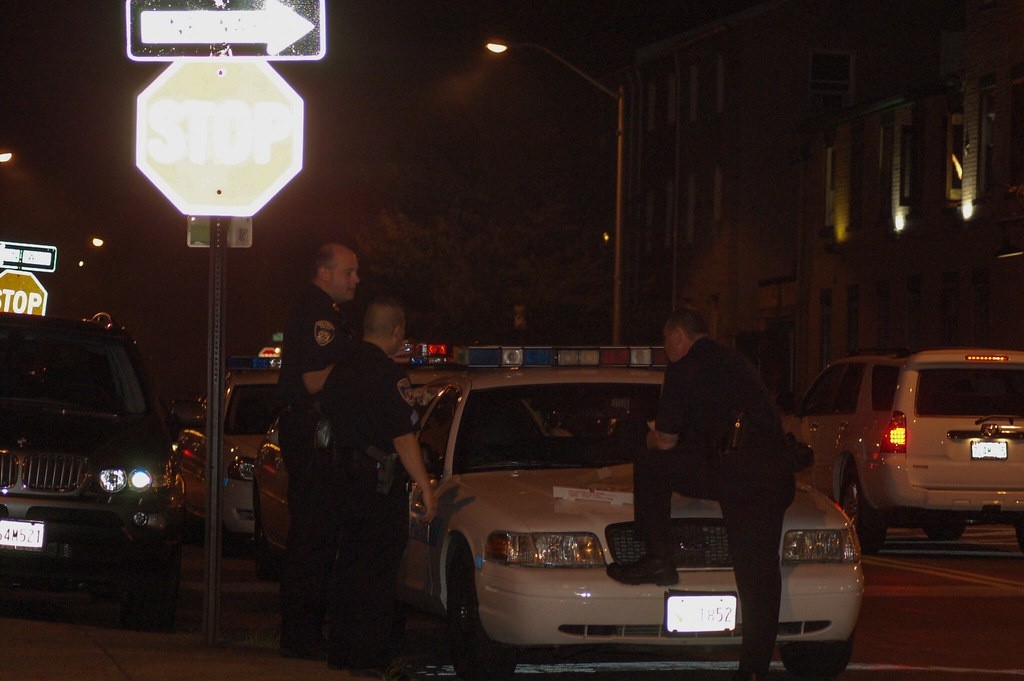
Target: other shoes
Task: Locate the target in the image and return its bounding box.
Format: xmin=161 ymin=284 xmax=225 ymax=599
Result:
xmin=278 ymin=646 xmax=329 ymax=661
xmin=326 ymin=660 xmax=344 ymax=669
xmin=606 ymin=554 xmax=679 ymax=586
xmin=348 ymin=661 xmax=392 ymax=677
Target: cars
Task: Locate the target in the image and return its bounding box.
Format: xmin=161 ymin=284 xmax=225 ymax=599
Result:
xmin=402 ymin=347 xmax=863 ymax=670
xmin=5 ymin=317 xmax=183 ymax=623
xmin=185 ymin=350 xmax=291 ymax=546
xmin=786 ymin=352 xmax=1021 ymax=554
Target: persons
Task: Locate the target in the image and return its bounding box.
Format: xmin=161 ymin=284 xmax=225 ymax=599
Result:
xmin=274 ymin=243 xmax=411 ymax=661
xmin=606 ymin=308 xmax=796 ymax=681
xmin=318 ymin=303 xmax=437 ymax=681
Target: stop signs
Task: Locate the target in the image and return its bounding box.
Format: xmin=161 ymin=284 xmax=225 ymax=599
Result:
xmin=0 ymin=271 xmax=49 ymax=316
xmin=135 ymin=63 xmax=303 ymax=219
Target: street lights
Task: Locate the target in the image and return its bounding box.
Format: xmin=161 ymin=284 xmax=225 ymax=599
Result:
xmin=484 ymin=39 xmax=627 ymax=351
xmin=2 ymin=150 xmax=26 ymax=241
xmin=90 ymin=232 xmax=152 ymax=317
